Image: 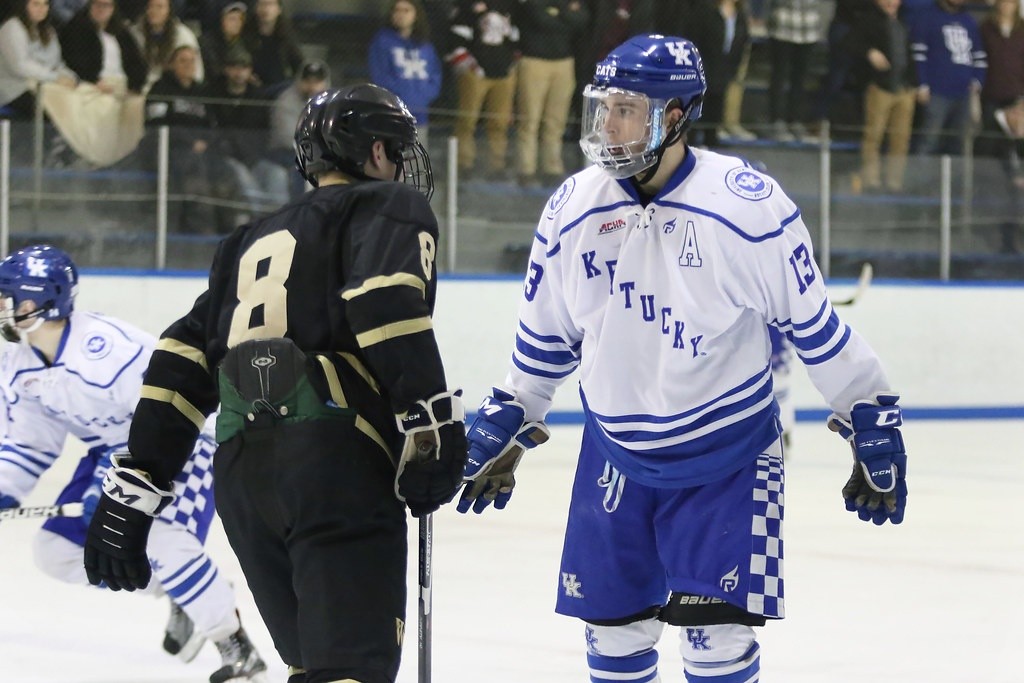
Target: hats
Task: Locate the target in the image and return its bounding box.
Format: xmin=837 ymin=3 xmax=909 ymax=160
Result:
xmin=221 ymin=2 xmax=248 ymax=15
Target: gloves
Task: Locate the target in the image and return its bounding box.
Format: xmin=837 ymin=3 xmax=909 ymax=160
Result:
xmin=84 ymin=453 xmax=177 ymax=592
xmin=395 ymin=390 xmax=471 ymax=518
xmin=445 ymin=386 xmax=550 ymax=514
xmin=826 ymin=392 xmax=909 ymax=525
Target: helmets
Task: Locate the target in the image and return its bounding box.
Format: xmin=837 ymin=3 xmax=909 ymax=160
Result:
xmin=292 ymin=84 xmax=435 ymax=203
xmin=577 ymin=34 xmax=708 ymax=179
xmin=0 ymin=245 xmax=79 ymax=343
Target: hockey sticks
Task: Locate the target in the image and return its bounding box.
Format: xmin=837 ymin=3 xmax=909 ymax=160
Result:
xmin=0 ymin=502 xmax=84 ymax=522
xmin=417 ymin=513 xmax=432 ymax=683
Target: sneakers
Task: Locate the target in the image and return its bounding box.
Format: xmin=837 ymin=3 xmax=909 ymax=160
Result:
xmin=209 ymin=610 xmax=268 ymax=683
xmin=162 ymin=598 xmax=205 ymax=664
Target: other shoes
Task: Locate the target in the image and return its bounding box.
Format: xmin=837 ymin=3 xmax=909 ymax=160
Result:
xmin=543 ymin=172 xmax=570 ymax=189
xmin=714 ymin=124 xmax=758 ymax=144
xmin=766 ymin=121 xmax=795 ymax=144
xmin=45 ymin=146 xmax=85 ymax=172
xmin=457 ymin=163 xmax=485 ymax=179
xmin=788 ymin=122 xmax=818 ymax=144
xmin=518 ymin=172 xmax=541 ymax=191
xmin=485 ymin=170 xmax=512 ymax=186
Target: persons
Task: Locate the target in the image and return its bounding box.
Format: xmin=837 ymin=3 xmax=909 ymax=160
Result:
xmin=1 ymin=0 xmax=333 ymax=238
xmin=762 ymin=1 xmax=825 ymax=146
xmin=450 ymin=32 xmax=909 ymax=683
xmin=850 ymin=0 xmax=920 ymax=196
xmin=80 ymin=77 xmax=467 ymax=683
xmin=1 ymin=242 xmax=268 ymax=683
xmin=902 ymin=0 xmax=991 ymax=185
xmin=980 ymin=0 xmax=1024 ymax=185
xmin=437 ymin=1 xmax=756 ymax=188
xmin=366 ymin=0 xmax=443 ymax=171
